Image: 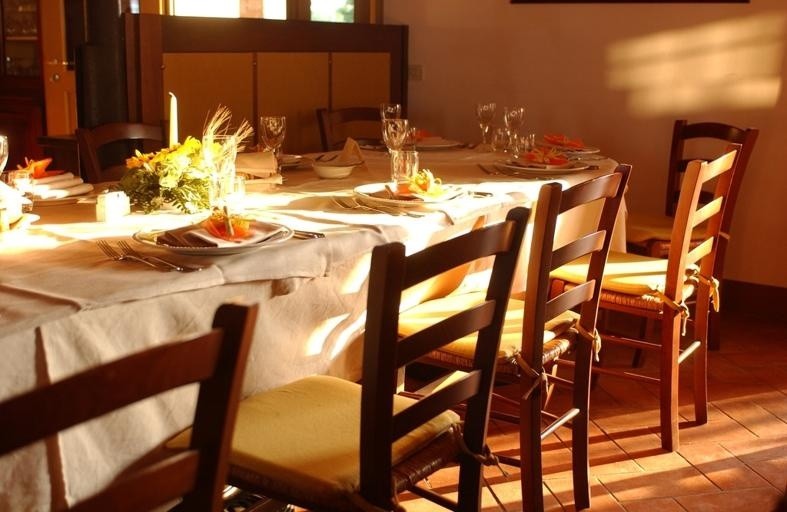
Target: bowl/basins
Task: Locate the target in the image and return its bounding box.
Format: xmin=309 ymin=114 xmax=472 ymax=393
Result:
xmin=311 ymin=165 xmax=354 ymax=179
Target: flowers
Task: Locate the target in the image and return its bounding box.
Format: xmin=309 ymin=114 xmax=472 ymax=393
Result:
xmin=104 ymin=101 xmax=256 ymax=217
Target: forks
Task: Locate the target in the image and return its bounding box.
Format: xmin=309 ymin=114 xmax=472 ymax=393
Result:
xmin=330 ymin=195 xmax=423 ymax=218
xmin=115 ymin=240 xmax=186 ymax=271
xmin=475 ymin=164 xmax=563 ymax=181
xmin=96 ymin=239 xmax=171 ymax=272
xmin=313 ymin=154 xmax=338 ymax=163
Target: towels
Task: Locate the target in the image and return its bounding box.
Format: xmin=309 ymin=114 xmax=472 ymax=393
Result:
xmin=233 ymin=149 xmax=280 ymax=180
xmin=409 ymin=128 xmax=437 ymax=144
xmin=12 ymin=156 xmax=68 ymax=180
xmin=543 ymin=132 xmax=585 ymax=151
xmin=521 ymin=145 xmax=569 ymax=167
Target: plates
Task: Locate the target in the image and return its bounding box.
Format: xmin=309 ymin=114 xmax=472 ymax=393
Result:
xmin=533 ymin=141 xmax=600 ymax=155
xmin=495 ymin=160 xmax=587 ymax=172
xmin=132 ymin=220 xmax=294 ymax=255
xmin=280 ymin=155 xmax=313 ymax=168
xmin=33 ymin=195 xmax=85 ymax=207
xmin=351 ymin=182 xmax=458 ymax=204
xmin=417 ymin=139 xmax=462 ymax=150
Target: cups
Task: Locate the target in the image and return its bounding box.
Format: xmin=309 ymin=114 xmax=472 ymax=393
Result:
xmin=225 ymin=176 xmax=246 ymax=213
xmin=0 ymin=133 xmax=10 ymax=178
xmin=8 ymin=170 xmax=36 ymax=214
xmin=203 ymin=135 xmax=237 ymax=207
xmin=379 ymin=102 xmax=420 ymax=181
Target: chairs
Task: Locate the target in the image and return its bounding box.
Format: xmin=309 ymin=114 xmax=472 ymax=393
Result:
xmin=72 ymin=119 xmax=172 ymax=186
xmin=34 ymin=134 xmax=81 ymax=181
xmin=0 ymin=296 xmax=264 ymax=511
xmin=595 ymin=117 xmax=760 ymax=369
xmin=314 ymin=102 xmax=404 ymax=155
xmin=392 ymin=161 xmax=633 ymax=512
xmin=166 ymin=202 xmax=535 ymax=512
xmin=528 ymin=142 xmax=742 ymax=454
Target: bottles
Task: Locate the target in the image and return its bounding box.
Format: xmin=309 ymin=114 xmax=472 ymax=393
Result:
xmin=95 ymin=191 xmax=131 ymax=222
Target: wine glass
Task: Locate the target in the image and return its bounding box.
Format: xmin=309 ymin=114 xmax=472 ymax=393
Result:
xmin=473 ymin=101 xmax=534 ymax=158
xmin=259 ymin=116 xmax=288 ymax=181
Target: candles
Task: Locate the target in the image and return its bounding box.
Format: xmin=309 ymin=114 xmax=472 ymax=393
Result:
xmin=167 ymin=90 xmax=179 ymax=150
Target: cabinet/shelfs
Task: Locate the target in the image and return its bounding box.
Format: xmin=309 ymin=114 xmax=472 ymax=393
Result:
xmin=0 ymin=0 xmax=40 ymax=99
xmin=0 ymin=92 xmax=45 ymax=174
